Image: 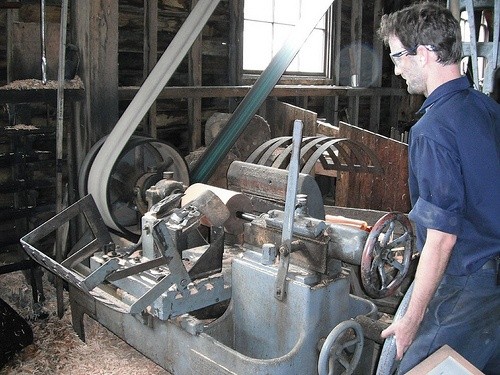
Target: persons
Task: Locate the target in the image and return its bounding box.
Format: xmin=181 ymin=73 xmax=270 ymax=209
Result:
xmin=375 ymin=1 xmax=499 ymax=374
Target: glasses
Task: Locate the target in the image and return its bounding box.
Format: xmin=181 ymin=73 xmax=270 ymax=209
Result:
xmin=389 ymin=43 xmax=435 ymax=67
